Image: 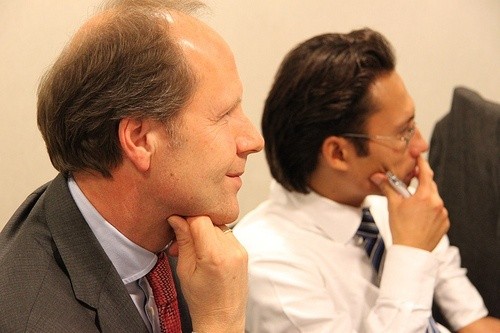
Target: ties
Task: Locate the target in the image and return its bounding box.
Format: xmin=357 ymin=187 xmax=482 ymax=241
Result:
xmin=361 ymin=208 xmax=440 ymax=332
xmin=148 ymin=251 xmax=181 ymax=332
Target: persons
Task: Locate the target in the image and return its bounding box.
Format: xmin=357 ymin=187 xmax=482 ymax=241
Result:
xmin=229 ymin=25 xmax=500 ymax=332
xmin=0 ymin=0 xmax=266 ymax=333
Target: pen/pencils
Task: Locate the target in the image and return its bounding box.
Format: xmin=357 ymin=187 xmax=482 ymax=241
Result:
xmin=386 ymin=171 xmax=413 ymax=200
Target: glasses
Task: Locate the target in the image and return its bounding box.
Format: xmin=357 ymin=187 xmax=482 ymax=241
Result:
xmin=344 ymin=123 xmax=417 ymax=146
